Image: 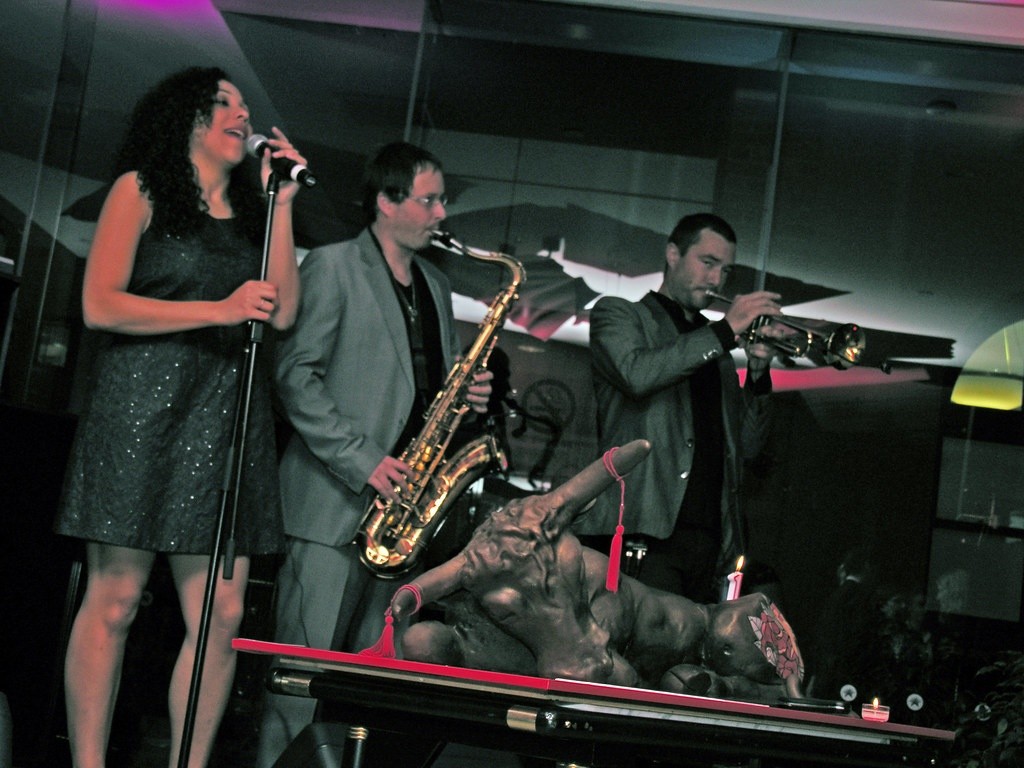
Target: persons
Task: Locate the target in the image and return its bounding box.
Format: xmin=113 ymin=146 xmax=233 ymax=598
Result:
xmin=589 ymin=212 xmax=785 ymax=604
xmin=257 ymin=143 xmax=493 ymax=768
xmin=56 ymin=67 xmax=307 ymax=768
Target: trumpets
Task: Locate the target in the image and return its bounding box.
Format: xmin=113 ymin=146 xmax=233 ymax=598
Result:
xmin=706 ymin=287 xmax=867 ymax=372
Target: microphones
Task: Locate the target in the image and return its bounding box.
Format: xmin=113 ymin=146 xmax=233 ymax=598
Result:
xmin=245 ymin=134 xmax=318 ymax=188
xmin=880 ymin=360 xmax=890 ymax=376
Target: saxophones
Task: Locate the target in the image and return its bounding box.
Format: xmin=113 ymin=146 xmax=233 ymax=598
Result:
xmin=355 ymin=229 xmax=529 ymax=580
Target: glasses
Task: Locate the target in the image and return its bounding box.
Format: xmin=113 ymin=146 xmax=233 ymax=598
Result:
xmin=406 ymin=195 xmax=448 ymax=208
